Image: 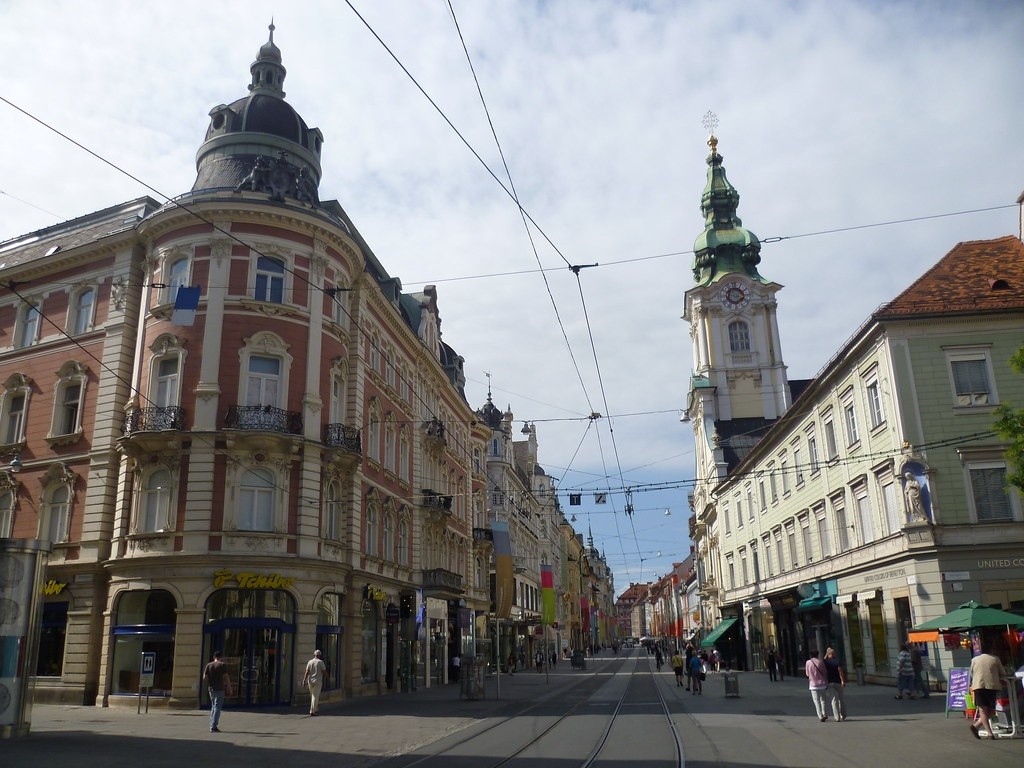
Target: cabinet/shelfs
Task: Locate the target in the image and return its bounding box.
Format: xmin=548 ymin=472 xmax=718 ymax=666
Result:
xmin=978 ymin=676 xmax=1024 ymax=739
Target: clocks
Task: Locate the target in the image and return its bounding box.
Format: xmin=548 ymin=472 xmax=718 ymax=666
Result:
xmin=720 ymin=282 xmax=751 ymax=310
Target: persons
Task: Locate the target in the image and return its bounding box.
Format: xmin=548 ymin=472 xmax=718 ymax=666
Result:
xmin=805 ymin=649 xmax=828 ymax=722
xmin=450 ymin=656 xmax=459 ymax=681
xmin=514 ymin=646 xmax=526 ymax=670
xmin=689 ymin=651 xmax=702 ymax=695
xmin=1014 ymin=663 xmax=1024 ymax=687
xmin=763 ymin=635 xmax=777 ymax=669
xmin=905 ymin=641 xmax=929 ymax=698
xmin=709 ymin=650 xmax=716 ymax=673
xmin=776 ymin=655 xmax=785 ymax=680
xmin=713 ymin=648 xmax=723 ymax=672
xmin=967 ymin=646 xmax=1005 ymax=740
xmin=202 ymin=650 xmax=232 ymax=733
xmin=533 ymin=625 xmax=699 ymax=674
xmin=507 ymin=653 xmax=511 ymax=676
xmin=701 ymin=649 xmax=708 ymax=673
xmin=764 ymin=645 xmax=777 ymax=681
xmin=893 ymin=644 xmax=914 ymax=699
xmin=685 ymin=649 xmax=698 ymax=692
xmin=303 ymin=650 xmax=330 ymax=714
xmin=824 ymin=646 xmax=846 ymax=722
xmin=672 ymin=651 xmax=683 ymax=686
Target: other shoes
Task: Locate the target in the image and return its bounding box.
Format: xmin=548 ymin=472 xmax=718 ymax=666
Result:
xmin=894 ymin=695 xmax=902 ymax=699
xmin=985 ymin=736 xmax=995 ymax=740
xmin=695 ymin=688 xmax=698 ymax=691
xmin=907 ymin=697 xmax=914 ymax=699
xmin=680 ymin=681 xmax=683 ymax=686
xmin=920 ymin=695 xmax=930 ymax=698
xmin=677 ymin=684 xmax=679 ymax=686
xmin=210 ymin=727 xmax=220 ymax=733
xmin=906 ymin=692 xmax=911 ymax=696
xmin=311 ymin=712 xmax=319 ymax=716
xmin=820 ymin=714 xmax=828 ymax=721
xmin=685 ymin=688 xmax=690 ymax=691
xmin=692 ymin=693 xmax=695 ymax=695
xmin=835 ymin=715 xmax=845 ymax=722
xmin=698 ymin=693 xmax=701 ymax=695
xmin=969 ymin=726 xmax=980 ymax=739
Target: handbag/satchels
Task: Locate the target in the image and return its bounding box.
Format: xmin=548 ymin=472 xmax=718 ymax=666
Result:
xmin=699 ymin=672 xmax=705 ymax=681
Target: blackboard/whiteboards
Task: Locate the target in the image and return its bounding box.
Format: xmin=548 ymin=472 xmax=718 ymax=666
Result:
xmin=945 ymin=668 xmax=972 ymax=711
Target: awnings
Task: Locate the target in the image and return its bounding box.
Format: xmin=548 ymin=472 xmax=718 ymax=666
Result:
xmin=701 ymin=619 xmax=739 ymax=647
xmin=795 ymin=599 xmax=832 ymax=613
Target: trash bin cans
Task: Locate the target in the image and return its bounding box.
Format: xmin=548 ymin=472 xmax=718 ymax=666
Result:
xmin=569 ymin=650 xmax=587 ymax=669
xmin=459 ymin=656 xmax=486 ymax=701
xmin=722 ymin=672 xmax=740 ymax=698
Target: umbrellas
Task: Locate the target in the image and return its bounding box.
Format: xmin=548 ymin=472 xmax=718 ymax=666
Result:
xmin=909 ymin=601 xmax=1024 ymax=671
